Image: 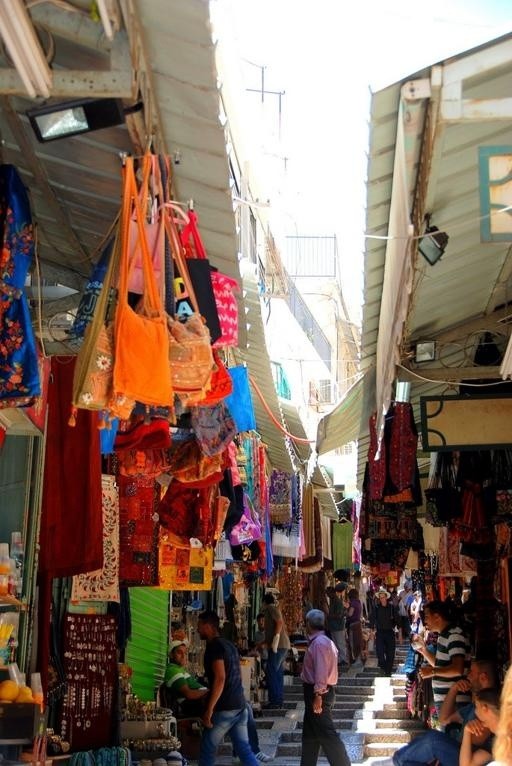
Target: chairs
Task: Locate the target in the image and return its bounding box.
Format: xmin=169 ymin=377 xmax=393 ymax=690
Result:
xmin=159 ymin=685 xmax=204 ymax=744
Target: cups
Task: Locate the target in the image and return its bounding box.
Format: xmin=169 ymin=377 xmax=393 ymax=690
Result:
xmin=6 ymin=662 xmax=44 ymax=697
xmin=0 ymin=531 xmax=24 ymax=598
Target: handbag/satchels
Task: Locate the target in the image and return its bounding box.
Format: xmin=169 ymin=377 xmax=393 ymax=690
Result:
xmin=67 ymin=152 xmax=256 ymax=435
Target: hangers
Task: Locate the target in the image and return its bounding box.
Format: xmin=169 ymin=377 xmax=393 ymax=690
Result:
xmin=384 ymin=400 xmax=397 ymax=420
xmin=339 ymin=513 xmax=350 ymax=522
xmin=130 ymin=195 xmax=193 ymax=211
xmin=250 ymin=429 xmax=261 ymax=440
xmin=118 ymin=134 xmax=179 ymax=166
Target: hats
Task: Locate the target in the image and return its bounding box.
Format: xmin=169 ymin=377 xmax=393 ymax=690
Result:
xmin=375 ymin=587 xmax=391 ymax=599
xmin=168 ymin=640 xmax=189 ymax=656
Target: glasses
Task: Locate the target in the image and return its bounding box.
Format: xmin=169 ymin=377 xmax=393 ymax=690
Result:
xmin=414 ymin=595 xmax=417 ymax=596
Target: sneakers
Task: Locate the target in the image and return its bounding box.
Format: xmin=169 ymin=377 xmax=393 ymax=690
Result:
xmin=255 ymin=752 xmax=273 ymax=762
xmin=232 ymin=757 xmax=241 ymax=764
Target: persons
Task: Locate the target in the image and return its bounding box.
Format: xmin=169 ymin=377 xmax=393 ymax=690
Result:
xmin=252 ymin=612 xmax=268 ymax=691
xmin=265 ymin=593 xmax=291 ymax=707
xmin=165 ymin=641 xmax=273 ymax=762
xmin=487 ymin=664 xmax=512 ymax=766
xmin=300 ymin=609 xmax=351 ymax=765
xmin=459 ymin=688 xmax=500 ymax=766
xmin=328 ymin=582 xmax=424 ymax=677
xmin=413 ymin=600 xmax=474 ymax=719
xmin=370 ymin=658 xmax=500 ymax=766
xmin=198 ymin=610 xmax=260 ymax=765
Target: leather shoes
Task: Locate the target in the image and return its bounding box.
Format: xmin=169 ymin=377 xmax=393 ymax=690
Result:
xmin=261 ymin=702 xmax=282 ymax=709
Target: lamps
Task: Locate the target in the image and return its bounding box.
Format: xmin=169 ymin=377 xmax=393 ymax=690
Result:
xmin=26 ymin=97 xmax=126 ymax=144
xmin=417 ymin=213 xmax=450 ymax=266
xmin=414 ymin=338 xmax=436 ymax=364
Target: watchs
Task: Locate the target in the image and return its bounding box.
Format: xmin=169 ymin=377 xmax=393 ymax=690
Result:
xmin=432 ymin=667 xmax=436 ymax=675
xmin=313 ymin=693 xmax=322 ymax=697
